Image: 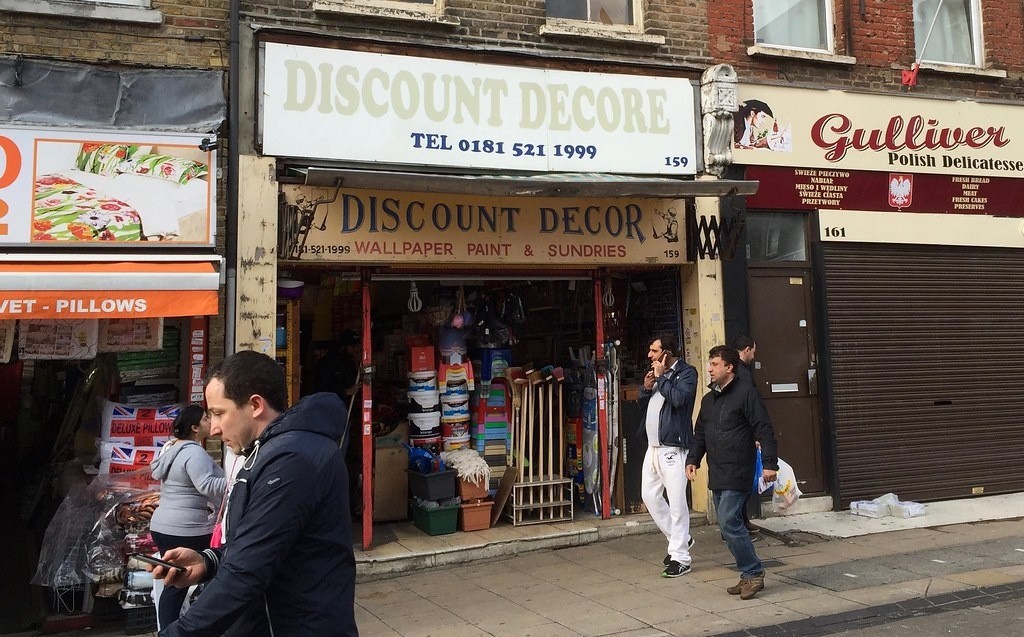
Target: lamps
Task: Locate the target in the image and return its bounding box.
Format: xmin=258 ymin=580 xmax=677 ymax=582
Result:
xmin=199 ymin=138 xmax=220 ymax=152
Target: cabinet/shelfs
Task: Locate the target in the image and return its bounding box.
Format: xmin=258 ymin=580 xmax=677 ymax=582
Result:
xmin=276 ymin=298 xmax=300 ymax=408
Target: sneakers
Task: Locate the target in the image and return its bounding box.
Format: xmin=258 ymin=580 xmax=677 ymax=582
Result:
xmin=726 ymin=579 xmax=765 ymax=594
xmin=661 ymin=561 xmax=691 ymax=577
xmin=740 ymin=569 xmax=765 ymax=599
xmin=662 ymin=538 xmax=695 ymax=566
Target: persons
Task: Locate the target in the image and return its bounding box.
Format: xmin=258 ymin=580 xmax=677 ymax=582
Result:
xmin=733 ymin=100 xmax=773 ymax=150
xmin=146 ymin=350 xmax=360 ymax=637
xmin=721 ymin=334 xmax=761 ymax=542
xmin=314 ymin=330 xmax=358 ymax=523
xmin=684 ymin=345 xmax=779 ymax=600
xmin=148 ymin=406 xmax=230 ymax=630
xmin=634 ymin=336 xmax=699 ymax=577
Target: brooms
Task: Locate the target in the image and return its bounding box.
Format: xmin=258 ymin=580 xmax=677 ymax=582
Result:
xmin=504 ymin=361 xmax=567 ymax=522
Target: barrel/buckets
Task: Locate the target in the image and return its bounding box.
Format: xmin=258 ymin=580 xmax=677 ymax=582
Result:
xmin=408 ymin=370 xmax=471 ymax=455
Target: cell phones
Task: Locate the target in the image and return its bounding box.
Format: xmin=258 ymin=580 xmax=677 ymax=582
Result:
xmin=136 ymin=555 xmax=187 ymax=572
xmin=657 ymin=349 xmax=673 ymax=365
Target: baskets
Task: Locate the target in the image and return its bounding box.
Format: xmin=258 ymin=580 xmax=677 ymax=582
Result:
xmin=119 ymin=607 xmax=157 ymax=635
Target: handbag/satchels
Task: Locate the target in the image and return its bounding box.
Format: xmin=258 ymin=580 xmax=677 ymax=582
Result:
xmin=753 ymin=447 xmax=764 ymax=494
xmin=773 ymin=456 xmax=803 ymax=512
xmin=210 ymin=522 xmax=224 ymax=549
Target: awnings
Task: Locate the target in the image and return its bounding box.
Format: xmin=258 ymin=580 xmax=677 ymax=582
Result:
xmin=289 ymin=166 xmax=760 ymax=198
xmin=0 ymin=261 xmax=220 ymax=319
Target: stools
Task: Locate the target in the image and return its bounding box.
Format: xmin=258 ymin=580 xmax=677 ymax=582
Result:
xmin=438 ymin=347 xmax=512 ymax=498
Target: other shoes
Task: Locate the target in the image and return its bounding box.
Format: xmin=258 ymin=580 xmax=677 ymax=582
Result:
xmin=721 ymin=532 xmax=758 ymax=543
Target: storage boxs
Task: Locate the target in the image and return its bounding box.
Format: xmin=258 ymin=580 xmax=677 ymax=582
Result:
xmin=410 ymin=345 xmax=435 ymax=372
xmin=457 ymin=475 xmax=488 ymax=500
xmin=124 ymin=608 xmax=157 ymax=636
xmin=404 ymin=468 xmax=457 ymax=501
xmin=408 ymin=498 xmax=460 ymax=536
xmin=458 ymin=501 xmax=495 ymax=531
